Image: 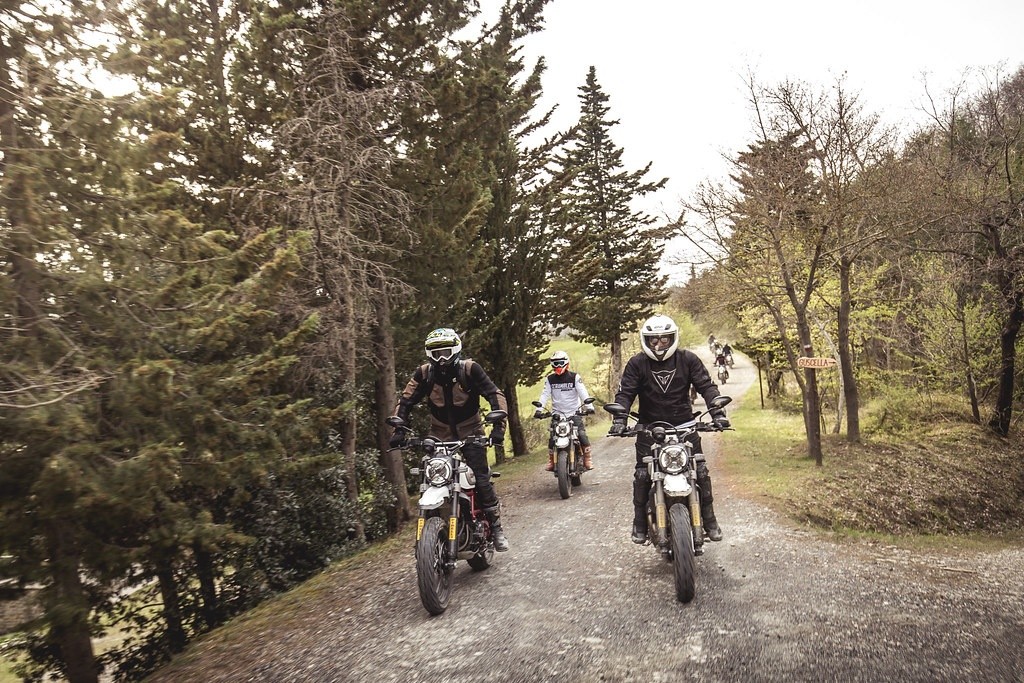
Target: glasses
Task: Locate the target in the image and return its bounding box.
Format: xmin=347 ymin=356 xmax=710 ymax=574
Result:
xmin=551 ymin=360 xmax=566 ymax=368
xmin=649 ymin=336 xmax=671 ymax=343
xmin=429 ymin=347 xmax=453 ymax=361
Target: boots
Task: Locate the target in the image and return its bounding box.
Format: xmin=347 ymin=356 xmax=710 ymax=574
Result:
xmin=582 ymin=445 xmax=593 ymax=469
xmin=481 ymin=498 xmax=508 ymax=551
xmin=697 ymin=477 xmax=722 ymax=541
xmin=632 ymin=480 xmax=653 ymax=543
xmin=547 ymin=452 xmax=553 ymax=470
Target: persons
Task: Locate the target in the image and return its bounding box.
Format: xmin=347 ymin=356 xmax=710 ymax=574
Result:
xmin=722 ymin=343 xmax=734 ymax=364
xmin=714 ymin=352 xmax=729 ymax=379
xmin=390 ymin=328 xmax=509 ymax=552
xmin=609 ymin=313 xmax=729 ymax=544
xmin=708 ymin=334 xmax=723 ymax=356
xmin=534 ymin=350 xmax=595 ymax=470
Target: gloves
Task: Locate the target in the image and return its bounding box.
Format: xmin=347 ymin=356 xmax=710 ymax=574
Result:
xmin=489 ymin=426 xmax=504 ymax=443
xmin=712 ymin=414 xmax=730 ymax=431
xmin=390 ymin=429 xmax=406 ymax=447
xmin=586 ymin=409 xmax=594 ymax=414
xmin=609 ymin=418 xmax=627 ymax=436
xmin=534 ymin=410 xmax=542 ymax=419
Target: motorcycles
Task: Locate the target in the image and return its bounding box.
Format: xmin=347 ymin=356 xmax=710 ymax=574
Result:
xmin=385 ymin=410 xmax=509 ymax=615
xmin=713 ymin=361 xmax=729 ymax=385
xmin=603 ymin=396 xmax=736 ymax=604
xmin=710 ymin=342 xmax=734 ymax=369
xmin=532 ymin=398 xmax=596 ymax=500
xmin=690 ymin=383 xmax=698 ymax=404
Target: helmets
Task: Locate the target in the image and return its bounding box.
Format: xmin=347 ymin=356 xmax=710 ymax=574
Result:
xmin=550 ymin=350 xmax=569 ymax=375
xmin=640 ymin=314 xmax=678 ymax=360
xmin=716 ymin=350 xmax=722 ymax=355
xmin=424 ymin=327 xmax=462 ymax=370
